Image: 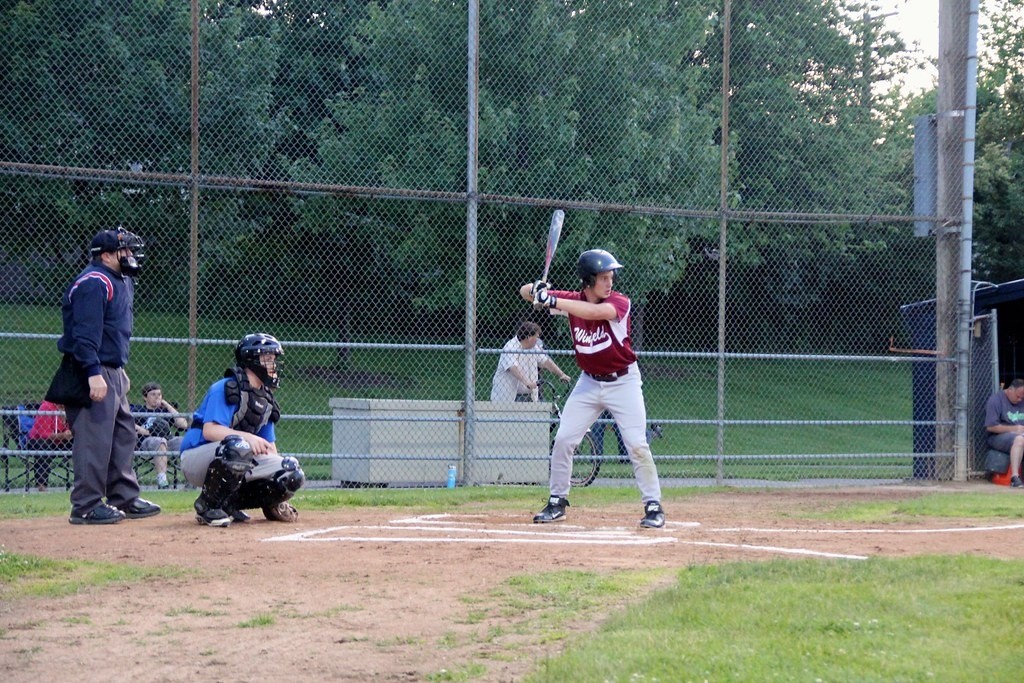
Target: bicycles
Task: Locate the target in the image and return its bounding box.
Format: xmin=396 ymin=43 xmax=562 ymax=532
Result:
xmin=517 ymin=378 xmax=601 ymax=488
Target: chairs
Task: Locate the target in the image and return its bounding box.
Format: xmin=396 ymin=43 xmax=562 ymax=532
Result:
xmin=2 ymin=402 xmax=188 ymax=493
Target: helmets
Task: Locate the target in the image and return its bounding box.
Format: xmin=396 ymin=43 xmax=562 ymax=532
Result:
xmin=235 ymin=332 xmax=284 ymax=388
xmin=576 ymin=249 xmax=624 ymax=288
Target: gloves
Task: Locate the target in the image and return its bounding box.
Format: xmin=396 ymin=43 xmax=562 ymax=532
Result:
xmin=533 ymin=287 xmax=557 ymax=308
xmin=530 ymin=280 xmax=551 ymax=296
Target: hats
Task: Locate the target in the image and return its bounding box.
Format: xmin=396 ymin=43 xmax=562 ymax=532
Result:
xmin=91 ymin=231 xmax=144 ymax=252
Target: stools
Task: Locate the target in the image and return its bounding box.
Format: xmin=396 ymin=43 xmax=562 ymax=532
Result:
xmin=992 ymin=459 xmax=1022 ymax=485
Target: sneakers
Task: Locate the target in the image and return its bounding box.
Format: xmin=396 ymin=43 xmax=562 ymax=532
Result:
xmin=157 ymin=475 xmax=169 ymax=489
xmin=223 ymin=503 xmax=249 ymax=521
xmin=640 ymin=500 xmax=665 ymax=529
xmin=194 ymin=498 xmax=230 ymax=527
xmin=1010 ymin=475 xmax=1023 ymax=488
xmin=107 ymin=497 xmax=161 ymax=519
xmin=533 ymin=495 xmax=570 ymax=523
xmin=69 ymin=503 xmax=126 ymax=524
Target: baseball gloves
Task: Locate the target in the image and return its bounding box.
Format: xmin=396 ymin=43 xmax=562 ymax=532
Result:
xmin=262 ymin=503 xmax=300 ymax=522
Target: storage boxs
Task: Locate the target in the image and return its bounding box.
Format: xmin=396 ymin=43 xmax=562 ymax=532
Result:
xmin=330 ymin=398 xmax=553 ymax=490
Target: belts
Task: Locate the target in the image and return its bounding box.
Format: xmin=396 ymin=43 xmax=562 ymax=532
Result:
xmin=99 ymin=360 xmax=124 ymax=369
xmin=584 ymin=368 xmax=628 ymax=382
xmin=517 ymin=393 xmax=530 ymax=397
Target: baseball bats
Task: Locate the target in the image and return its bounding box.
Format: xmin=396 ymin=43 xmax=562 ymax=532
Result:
xmin=533 ymin=209 xmax=565 ymax=311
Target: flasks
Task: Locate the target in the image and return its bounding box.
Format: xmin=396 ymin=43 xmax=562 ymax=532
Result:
xmin=447 ymin=463 xmax=456 ymax=489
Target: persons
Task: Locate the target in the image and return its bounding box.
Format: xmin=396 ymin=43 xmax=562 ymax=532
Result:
xmin=489 ymin=320 xmax=571 ymax=403
xmin=519 ymin=248 xmax=667 ymax=529
xmin=30 ymin=399 xmax=73 ymax=492
xmin=179 ymin=333 xmax=307 ymax=528
xmin=985 ymin=378 xmax=1024 ymax=489
xmin=44 ymin=228 xmax=164 ymax=526
xmin=132 ymin=381 xmax=199 ymax=491
